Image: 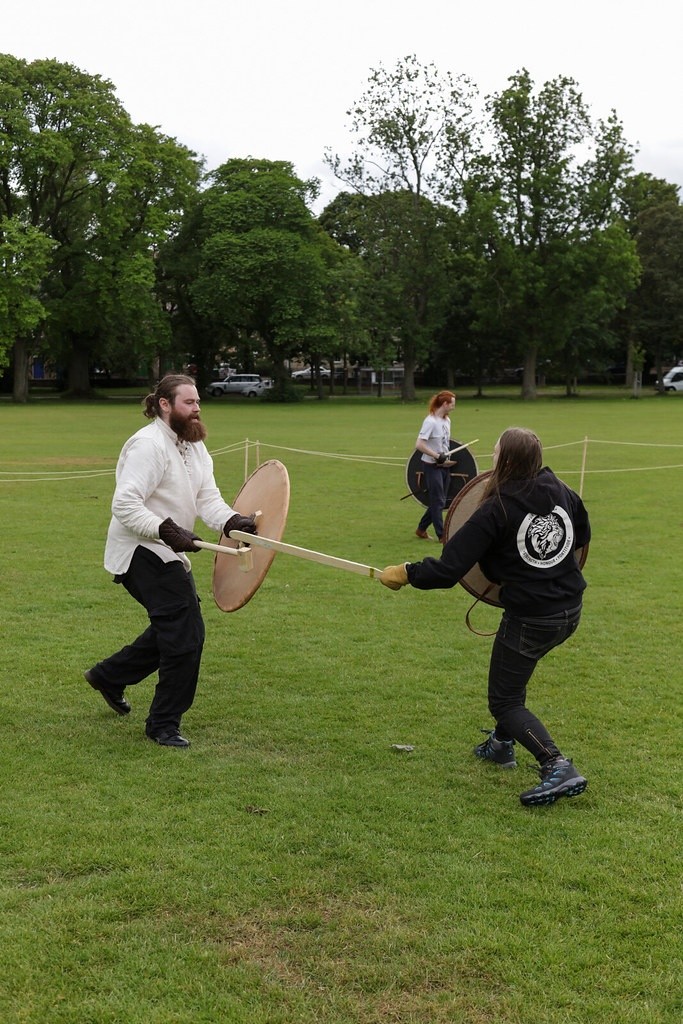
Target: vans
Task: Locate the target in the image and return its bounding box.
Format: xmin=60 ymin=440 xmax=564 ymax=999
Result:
xmin=655 ymin=367 xmax=683 ymax=393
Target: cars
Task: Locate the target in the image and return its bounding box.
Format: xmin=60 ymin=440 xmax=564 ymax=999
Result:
xmin=242 ymin=379 xmax=275 ymax=397
xmin=213 ymin=362 xmax=237 ymax=372
xmin=291 ymin=365 xmax=331 ymax=380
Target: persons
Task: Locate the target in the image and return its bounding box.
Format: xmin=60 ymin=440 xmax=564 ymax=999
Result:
xmin=416 ymin=390 xmax=456 ymax=543
xmin=379 ymin=428 xmax=592 ymax=806
xmin=86 ymin=374 xmax=257 ymax=746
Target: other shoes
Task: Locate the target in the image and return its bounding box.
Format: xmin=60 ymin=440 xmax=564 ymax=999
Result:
xmin=416 ymin=529 xmax=432 ymax=539
xmin=440 ymin=539 xmax=443 ymax=543
xmin=84 ymin=670 xmax=131 ymax=714
xmin=156 ymin=730 xmax=189 ymax=747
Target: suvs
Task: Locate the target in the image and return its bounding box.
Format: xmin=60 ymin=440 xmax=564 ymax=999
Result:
xmin=205 ymin=374 xmax=260 ymax=397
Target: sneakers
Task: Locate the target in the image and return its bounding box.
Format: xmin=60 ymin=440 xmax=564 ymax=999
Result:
xmin=476 ymin=733 xmax=517 ymax=767
xmin=519 ymin=759 xmax=588 ymax=806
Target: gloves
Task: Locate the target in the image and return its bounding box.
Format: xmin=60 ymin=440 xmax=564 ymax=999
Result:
xmin=436 ymin=452 xmax=448 ymax=464
xmin=381 ymin=562 xmax=412 ymax=590
xmin=159 ymin=517 xmax=202 ymax=553
xmin=223 ymin=515 xmax=256 ymax=546
